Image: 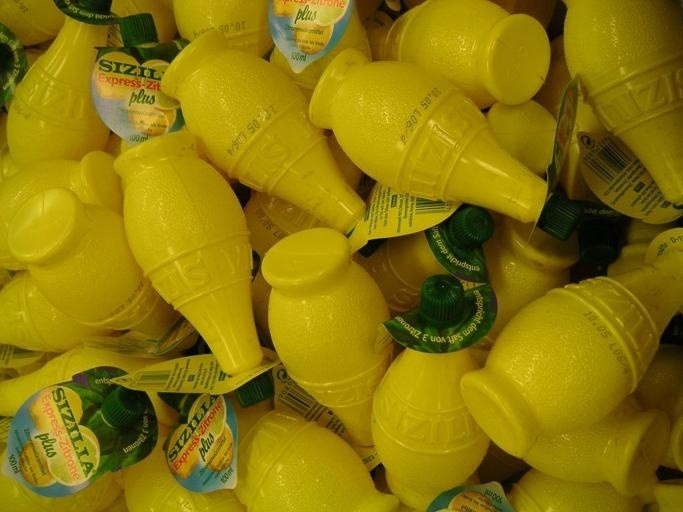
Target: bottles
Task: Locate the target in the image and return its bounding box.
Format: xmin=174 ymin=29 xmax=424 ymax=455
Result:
xmin=0 ymin=0 xmax=683 ymax=512
